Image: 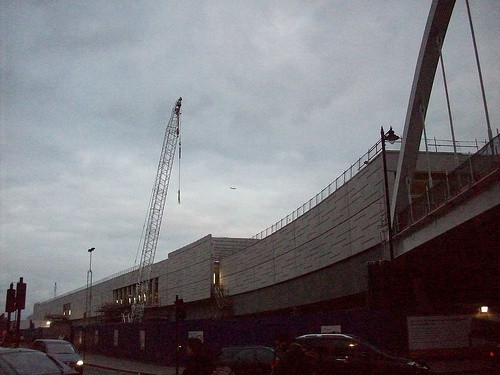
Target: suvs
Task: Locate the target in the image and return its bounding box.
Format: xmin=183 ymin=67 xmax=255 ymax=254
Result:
xmin=292 ymin=333 xmax=432 ymax=375
xmin=32 ymin=339 xmax=83 ymax=374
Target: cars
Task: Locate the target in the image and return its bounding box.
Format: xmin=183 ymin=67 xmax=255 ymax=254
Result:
xmin=1 ymin=348 xmax=80 ymax=375
xmin=214 ymin=347 xmax=277 ymax=375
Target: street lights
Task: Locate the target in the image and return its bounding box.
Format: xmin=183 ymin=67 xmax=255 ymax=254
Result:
xmin=84 ymin=248 xmax=96 ymax=316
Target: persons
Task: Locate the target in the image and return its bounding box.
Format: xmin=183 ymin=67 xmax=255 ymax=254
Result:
xmin=273 ymin=333 xmax=309 ymax=375
xmin=179 ymin=337 xmax=216 ymax=375
